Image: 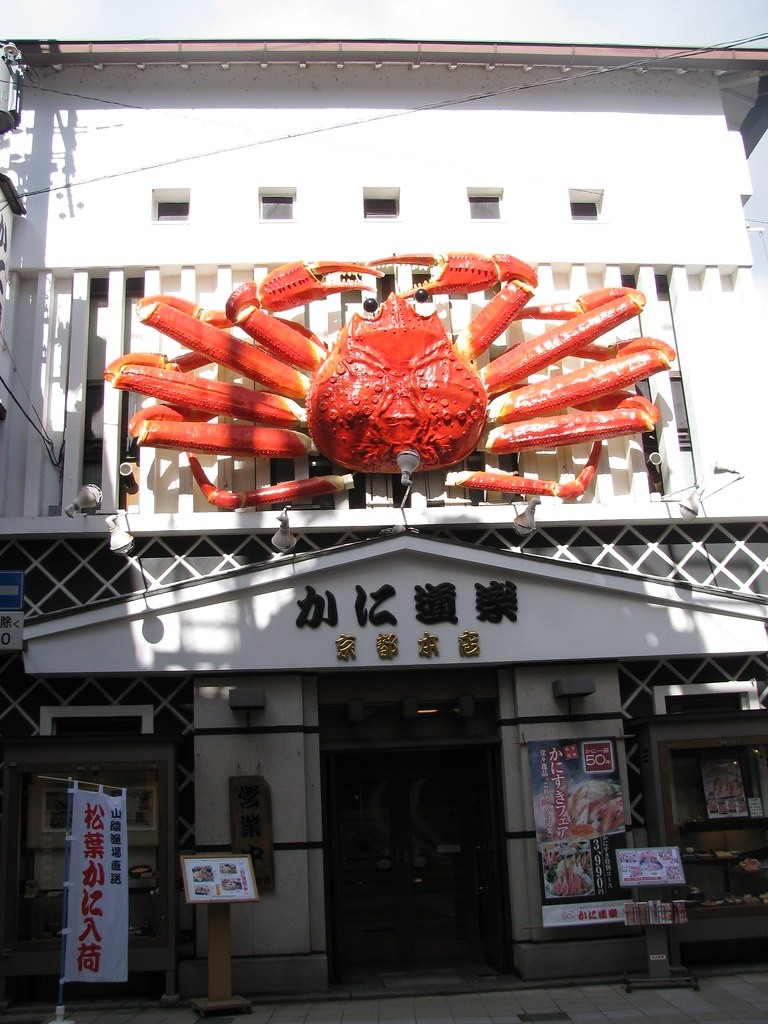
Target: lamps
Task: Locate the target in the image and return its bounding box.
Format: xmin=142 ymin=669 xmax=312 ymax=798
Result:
xmin=228 ymin=689 xmax=266 ymax=711
xmin=700 ymin=462 xmax=747 ymax=504
xmin=64 ymin=484 xmax=140 ymax=518
xmin=397 ymin=451 xmax=420 ymax=509
xmin=513 ymin=498 xmax=541 ymax=536
xmin=648 ymin=452 xmax=661 ymax=481
xmin=120 ymin=463 xmax=132 ymax=476
xmin=652 ymin=481 xmax=706 ymax=521
xmin=271 ymin=504 xmax=297 ymax=551
xmin=553 ymin=674 xmax=596 ymax=698
xmin=104 ymin=509 xmax=136 ymax=556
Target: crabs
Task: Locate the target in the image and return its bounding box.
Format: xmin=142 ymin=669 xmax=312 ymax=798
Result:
xmin=103 ymin=252 xmax=677 ymax=506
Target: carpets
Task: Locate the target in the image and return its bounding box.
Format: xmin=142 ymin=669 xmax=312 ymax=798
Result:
xmin=351 ymin=957 xmax=484 ymax=973
